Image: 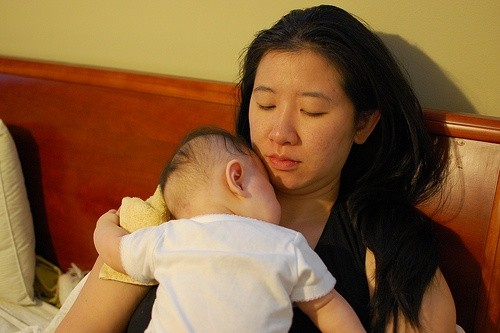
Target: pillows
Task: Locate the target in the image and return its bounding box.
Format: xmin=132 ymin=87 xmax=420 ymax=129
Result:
xmin=0 ymin=117 xmax=39 ymax=305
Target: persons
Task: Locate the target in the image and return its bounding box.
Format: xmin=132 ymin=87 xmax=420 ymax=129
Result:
xmin=53 ymin=4 xmax=458 ymax=333
xmin=92 ymin=128 xmax=369 ymax=333
